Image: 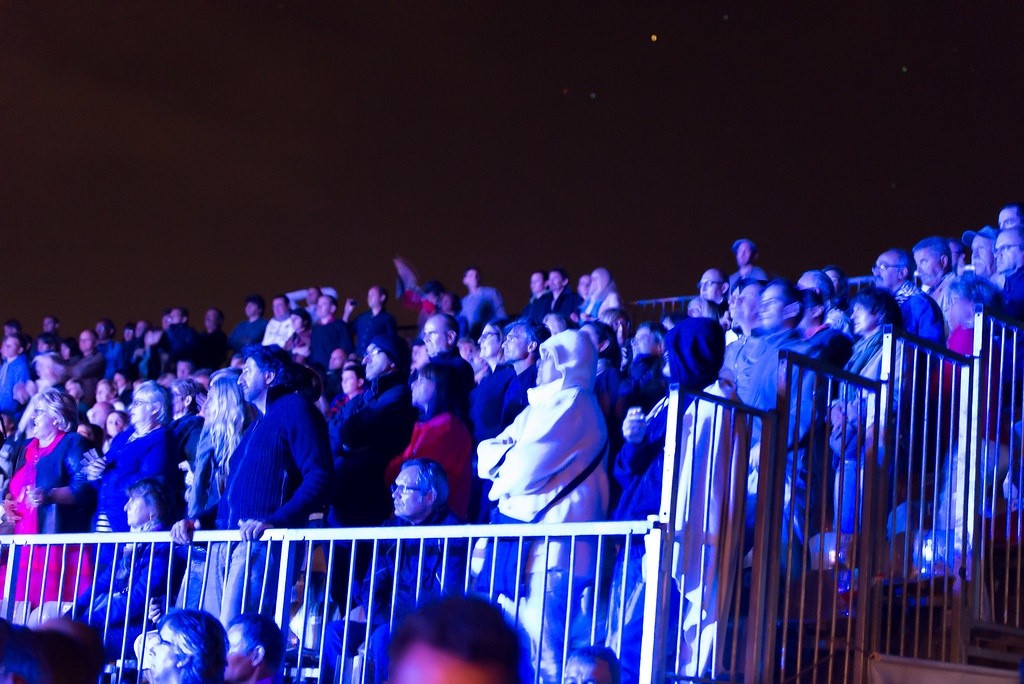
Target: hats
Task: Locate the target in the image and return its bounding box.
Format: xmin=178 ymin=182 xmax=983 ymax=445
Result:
xmin=962 ymin=225 xmax=1002 ymax=244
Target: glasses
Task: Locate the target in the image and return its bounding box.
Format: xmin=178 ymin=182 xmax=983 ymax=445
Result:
xmin=870 ymin=263 xmax=902 ymax=273
xmin=696 ymin=278 xmax=723 ymax=287
xmin=479 ymin=330 xmax=498 ymax=338
xmin=362 ymin=350 xmax=384 ymax=356
xmin=390 ymin=483 xmax=421 ymax=494
xmin=992 ymin=242 xmax=1019 ymax=257
xmin=154 ymin=634 xmax=175 ymax=648
xmin=132 ymin=398 xmax=147 ymax=406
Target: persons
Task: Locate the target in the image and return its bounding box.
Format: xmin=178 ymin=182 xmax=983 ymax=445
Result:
xmin=317 ymin=456 xmax=466 ymax=682
xmin=220 ymin=611 xmax=282 ymax=684
xmin=169 ymin=342 xmax=329 ymax=631
xmin=36 ymin=618 xmax=103 ymax=682
xmin=382 ymin=594 xmax=522 ymax=684
xmin=562 ymin=645 xmax=622 ymax=684
xmin=0 ymin=627 xmax=94 ymax=683
xmin=325 ymin=331 xmax=421 ymax=619
xmin=825 ymin=287 xmax=919 ymax=598
xmin=714 ymin=276 xmax=831 ymax=633
xmin=602 ymin=316 xmax=750 ymax=684
xmin=86 ymin=380 xmax=185 ymax=582
xmin=146 ymin=608 xmax=229 ymax=684
xmin=62 ymin=476 xmax=186 ymax=684
xmin=469 ymin=318 xmax=553 ymax=582
xmin=921 ymin=276 xmax=1014 ymax=631
xmin=167 ymin=377 xmax=206 ymax=530
xmin=1 ymin=205 xmax=1024 ymax=505
xmin=474 ymin=326 xmax=612 ymax=683
xmin=383 ymin=360 xmax=476 ymax=525
xmin=1 ymin=389 xmax=93 ymax=626
xmin=718 ymin=276 xmax=774 ymax=570
xmin=947 ymin=237 xmax=964 ymax=277
